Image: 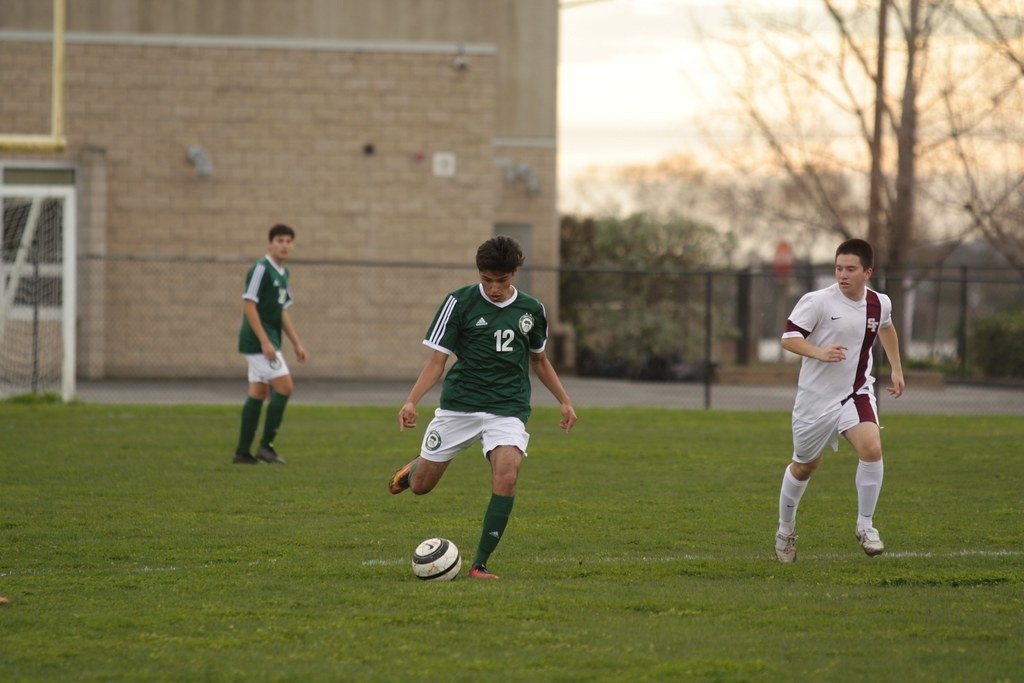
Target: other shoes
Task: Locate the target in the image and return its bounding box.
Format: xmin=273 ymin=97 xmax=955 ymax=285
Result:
xmin=256 ymin=444 xmax=284 ymax=465
xmin=232 ymin=452 xmax=259 ymax=465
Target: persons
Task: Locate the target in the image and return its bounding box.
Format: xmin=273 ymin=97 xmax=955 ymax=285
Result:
xmin=387 ymin=236 xmax=578 ymax=579
xmin=774 ymin=238 xmax=905 ymax=566
xmin=231 ymin=223 xmax=308 ymax=466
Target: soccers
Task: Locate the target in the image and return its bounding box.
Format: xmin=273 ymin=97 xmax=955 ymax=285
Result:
xmin=410 ymin=536 xmax=462 ymax=581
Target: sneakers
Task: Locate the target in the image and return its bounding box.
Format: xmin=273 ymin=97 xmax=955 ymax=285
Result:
xmin=775 ymin=523 xmax=798 ymax=563
xmin=855 ymin=525 xmax=885 ymax=556
xmin=388 ymin=454 xmax=421 ymax=495
xmin=470 ymin=565 xmax=499 ymax=580
